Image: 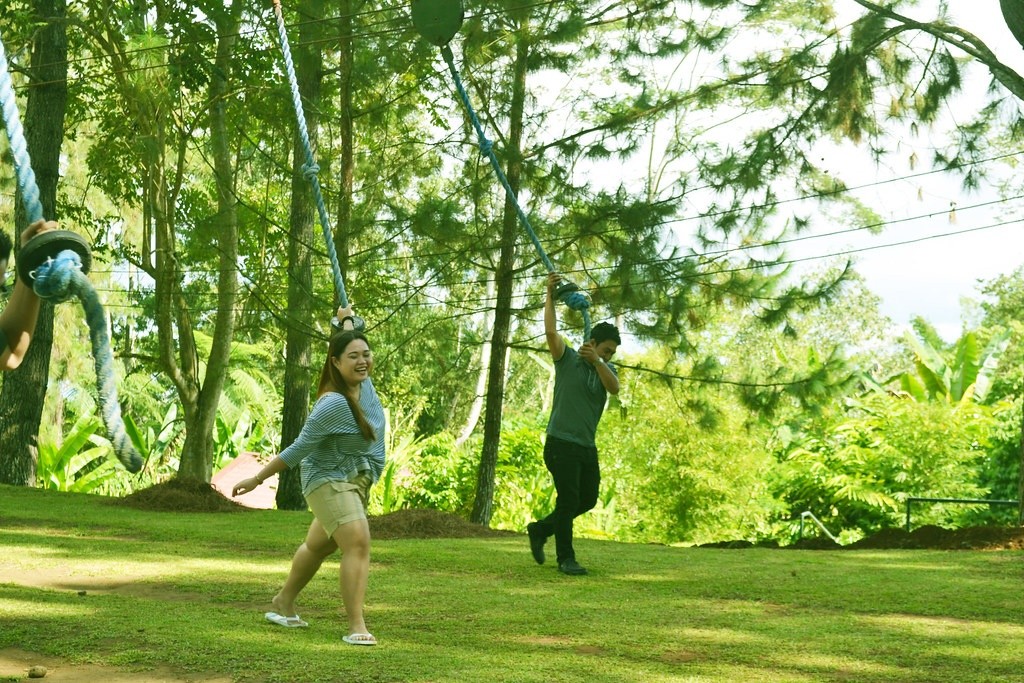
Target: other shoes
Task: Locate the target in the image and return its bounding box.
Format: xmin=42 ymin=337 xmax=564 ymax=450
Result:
xmin=558 ymin=558 xmax=586 ymax=575
xmin=527 ymin=522 xmax=545 ymax=565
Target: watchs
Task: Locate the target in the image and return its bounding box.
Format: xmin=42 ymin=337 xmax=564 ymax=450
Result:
xmin=593 ymin=357 xmax=604 ymax=366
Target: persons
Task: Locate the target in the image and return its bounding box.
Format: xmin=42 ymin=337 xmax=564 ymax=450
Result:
xmin=0 ymin=218 xmax=58 ymax=370
xmin=527 ymin=271 xmax=619 ymax=575
xmin=231 ymin=304 xmax=385 ymax=644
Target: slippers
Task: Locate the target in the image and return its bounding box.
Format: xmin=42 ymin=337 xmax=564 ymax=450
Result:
xmin=264 ymin=612 xmax=309 ymax=628
xmin=342 ymin=633 xmax=377 ymax=645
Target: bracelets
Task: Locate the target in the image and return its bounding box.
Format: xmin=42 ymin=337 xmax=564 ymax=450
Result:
xmin=341 ymin=316 xmax=355 ymax=328
xmin=255 ymin=474 xmax=263 ymax=485
